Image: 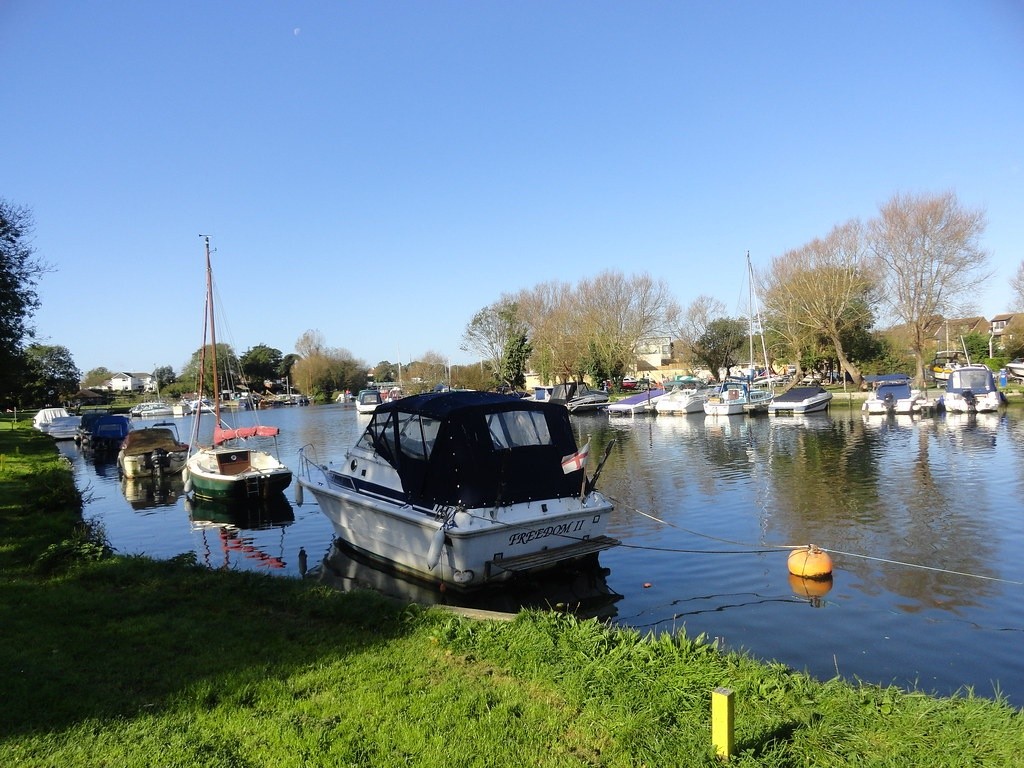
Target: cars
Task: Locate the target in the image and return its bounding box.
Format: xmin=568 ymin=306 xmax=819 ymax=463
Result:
xmin=595 ymin=349 xmax=1024 ymax=393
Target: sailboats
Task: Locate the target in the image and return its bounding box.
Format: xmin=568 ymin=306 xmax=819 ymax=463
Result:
xmin=182 ymin=230 xmax=293 ymax=500
xmin=702 ymin=250 xmax=773 ymax=417
xmin=183 ymin=495 xmax=295 ymax=575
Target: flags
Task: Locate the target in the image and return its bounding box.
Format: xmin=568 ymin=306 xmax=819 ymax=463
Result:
xmin=561 ymin=441 xmax=589 ymax=475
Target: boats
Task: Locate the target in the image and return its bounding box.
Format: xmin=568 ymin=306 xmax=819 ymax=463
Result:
xmin=295 ymin=386 xmax=622 ymax=590
xmin=119 ymin=426 xmax=191 ymax=476
xmin=32 ymin=384 xmax=402 ymax=467
xmin=298 ymin=528 xmax=623 ymax=630
xmin=767 ymin=385 xmax=833 ymax=417
xmin=935 ymin=366 xmax=1010 ymax=418
xmin=423 ymin=378 xmax=722 ymax=422
xmin=120 ymin=471 xmax=184 ymax=510
xmin=860 ymin=373 xmax=930 ymax=414
